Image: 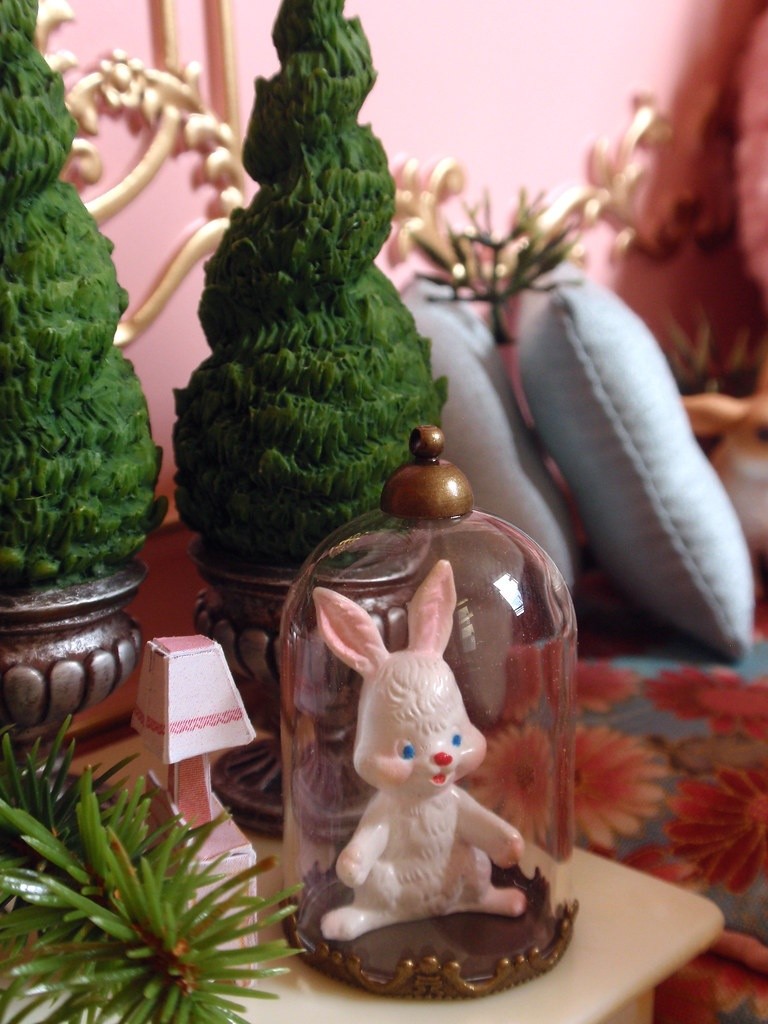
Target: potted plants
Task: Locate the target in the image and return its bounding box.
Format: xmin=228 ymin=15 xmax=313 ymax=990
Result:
xmin=167 ymin=0 xmax=453 ymax=839
xmin=0 ymin=0 xmax=162 ymax=737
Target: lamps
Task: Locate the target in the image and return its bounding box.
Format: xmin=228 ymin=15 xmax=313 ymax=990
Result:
xmin=132 ymin=635 xmax=260 ymax=995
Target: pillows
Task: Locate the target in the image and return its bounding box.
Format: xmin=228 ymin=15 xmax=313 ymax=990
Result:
xmin=515 ymin=259 xmax=757 ymax=661
xmin=385 ymin=274 xmax=575 ymax=610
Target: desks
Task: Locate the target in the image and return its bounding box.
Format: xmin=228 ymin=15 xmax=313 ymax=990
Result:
xmin=0 ymin=847 xmax=727 ymax=1024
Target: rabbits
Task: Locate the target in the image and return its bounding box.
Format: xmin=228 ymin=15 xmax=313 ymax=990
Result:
xmin=310 ymin=560 xmax=525 ymax=942
